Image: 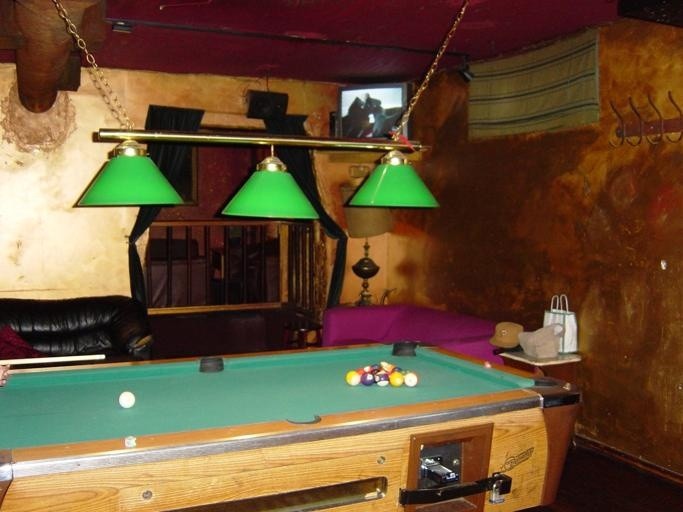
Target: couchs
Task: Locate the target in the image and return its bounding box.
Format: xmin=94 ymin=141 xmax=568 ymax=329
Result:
xmin=323 ymin=306 xmax=503 ymax=367
xmin=0 ymin=296 xmax=152 ymax=355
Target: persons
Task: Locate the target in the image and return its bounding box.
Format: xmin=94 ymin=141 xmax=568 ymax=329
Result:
xmin=0 ymin=363 xmax=10 ymax=386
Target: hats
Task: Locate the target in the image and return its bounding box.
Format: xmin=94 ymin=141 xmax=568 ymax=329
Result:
xmin=489 ymin=320 xmax=523 ymax=350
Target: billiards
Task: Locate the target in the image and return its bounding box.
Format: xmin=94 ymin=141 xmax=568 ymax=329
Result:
xmin=346 ymin=360 xmax=418 ymax=387
xmin=118 ymin=391 xmax=136 ymax=409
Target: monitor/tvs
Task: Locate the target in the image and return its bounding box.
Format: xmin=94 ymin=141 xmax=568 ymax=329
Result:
xmin=338 ymin=82 xmax=412 ymax=141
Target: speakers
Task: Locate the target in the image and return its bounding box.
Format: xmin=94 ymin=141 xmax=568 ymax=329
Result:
xmin=246 ymin=89 xmax=289 ymax=120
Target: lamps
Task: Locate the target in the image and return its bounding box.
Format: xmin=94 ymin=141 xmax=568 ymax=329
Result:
xmin=53 ymin=1 xmax=468 ymax=220
xmin=352 ymin=237 xmax=380 ymax=306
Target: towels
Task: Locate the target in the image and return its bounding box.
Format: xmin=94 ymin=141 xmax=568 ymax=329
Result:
xmin=463 ymin=28 xmax=599 ymax=139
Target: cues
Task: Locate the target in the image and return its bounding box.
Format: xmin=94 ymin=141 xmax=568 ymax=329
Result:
xmin=0 ymin=354 xmax=105 ymax=366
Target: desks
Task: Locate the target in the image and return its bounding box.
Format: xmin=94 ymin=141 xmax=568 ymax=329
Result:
xmin=1 ymin=339 xmax=580 ymax=512
xmin=500 ymin=350 xmax=582 ymax=376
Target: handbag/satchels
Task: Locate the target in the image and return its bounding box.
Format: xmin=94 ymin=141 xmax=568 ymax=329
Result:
xmin=519 ymin=323 xmax=566 ymax=359
xmin=542 ymin=293 xmax=579 ymax=354
